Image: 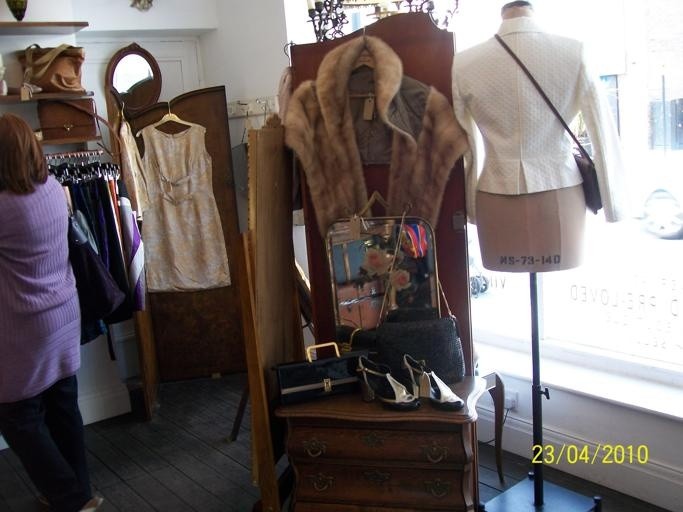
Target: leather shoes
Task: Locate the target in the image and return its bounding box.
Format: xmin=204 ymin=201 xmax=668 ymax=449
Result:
xmin=401 ymin=353 xmax=466 ymax=411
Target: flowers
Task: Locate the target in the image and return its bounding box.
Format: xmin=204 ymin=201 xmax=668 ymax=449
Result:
xmin=351 ymin=231 xmax=416 ymax=313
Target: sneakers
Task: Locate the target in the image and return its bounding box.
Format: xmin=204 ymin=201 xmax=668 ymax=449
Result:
xmin=81 ymin=495 xmax=105 ymax=512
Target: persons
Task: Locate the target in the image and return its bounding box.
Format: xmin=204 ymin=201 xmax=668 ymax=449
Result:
xmin=0 ymin=113 xmax=105 ymax=511
xmin=451 ymin=0 xmax=619 ymax=274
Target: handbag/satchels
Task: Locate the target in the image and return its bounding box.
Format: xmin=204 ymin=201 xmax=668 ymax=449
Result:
xmin=67 ymin=204 xmax=126 ymax=322
xmin=16 ymin=42 xmax=90 ymax=95
xmin=572 ymin=153 xmax=602 ymax=216
xmin=376 ymin=210 xmax=468 ymax=383
xmin=274 ymin=341 xmax=369 ymax=406
xmin=35 ymin=96 xmax=128 ymax=163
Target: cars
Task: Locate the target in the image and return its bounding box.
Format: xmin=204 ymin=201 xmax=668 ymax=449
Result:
xmin=627 ymin=147 xmax=682 ymax=242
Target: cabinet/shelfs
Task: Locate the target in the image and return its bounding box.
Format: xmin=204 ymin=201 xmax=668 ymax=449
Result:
xmin=0 ymin=19 xmax=101 ymax=145
xmin=274 ymin=365 xmax=510 ymax=512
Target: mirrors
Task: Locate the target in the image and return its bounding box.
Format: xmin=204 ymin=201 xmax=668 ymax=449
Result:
xmin=322 ymin=215 xmax=446 ymax=351
xmin=105 ymin=44 xmax=163 ymax=114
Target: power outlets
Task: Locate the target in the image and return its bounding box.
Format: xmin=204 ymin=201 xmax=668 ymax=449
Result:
xmin=503 ymin=387 xmax=520 ymax=415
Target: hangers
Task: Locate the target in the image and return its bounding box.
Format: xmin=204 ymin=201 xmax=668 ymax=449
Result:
xmin=339 ymin=23 xmax=380 ymax=101
xmin=44 ymin=148 xmax=120 ymax=179
xmin=134 ymin=99 xmax=207 ymax=138
xmin=283 ymin=42 xmax=297 ymax=68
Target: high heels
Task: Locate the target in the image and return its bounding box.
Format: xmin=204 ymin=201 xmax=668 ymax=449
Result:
xmin=354 ymin=356 xmax=422 ymax=410
